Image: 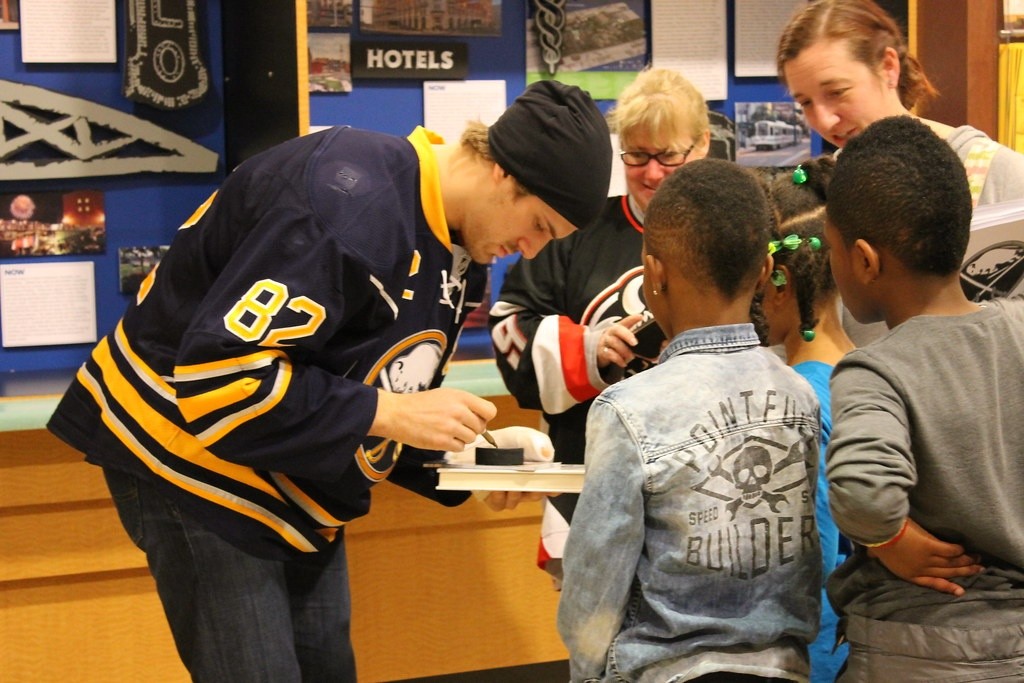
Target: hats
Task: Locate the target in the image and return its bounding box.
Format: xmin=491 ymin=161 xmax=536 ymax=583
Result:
xmin=488 ymin=79 xmax=613 ymax=231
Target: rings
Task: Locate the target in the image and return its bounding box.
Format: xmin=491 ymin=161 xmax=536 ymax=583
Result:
xmin=603 ymin=347 xmax=608 ymax=352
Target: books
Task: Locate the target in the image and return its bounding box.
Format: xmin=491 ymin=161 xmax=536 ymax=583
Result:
xmin=435 ymin=463 xmax=587 ymax=495
xmin=960 ymin=200 xmax=1023 ymax=305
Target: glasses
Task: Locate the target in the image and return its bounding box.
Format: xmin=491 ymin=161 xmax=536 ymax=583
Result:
xmin=620 ymin=132 xmax=704 ymax=167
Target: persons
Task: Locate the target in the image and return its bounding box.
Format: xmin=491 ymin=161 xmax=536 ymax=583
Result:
xmin=824 ymin=118 xmax=1024 ymax=683
xmin=775 ymin=0 xmax=1024 ymax=346
xmin=44 ymin=78 xmax=614 ymax=683
xmin=485 ymin=64 xmax=710 ymax=572
xmin=557 ymin=157 xmax=824 ymax=683
xmin=760 ymin=156 xmax=864 ymax=680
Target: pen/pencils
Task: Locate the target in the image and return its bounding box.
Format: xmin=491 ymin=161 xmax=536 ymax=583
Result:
xmin=482 ymin=431 xmax=498 ymax=448
